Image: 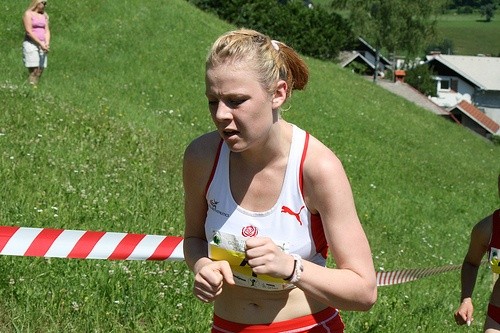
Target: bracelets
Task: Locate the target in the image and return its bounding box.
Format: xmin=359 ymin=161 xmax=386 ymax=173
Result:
xmin=290 ymin=254 xmax=303 ymax=284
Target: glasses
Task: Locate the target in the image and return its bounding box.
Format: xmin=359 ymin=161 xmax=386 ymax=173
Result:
xmin=41 ymin=2 xmax=46 ymax=5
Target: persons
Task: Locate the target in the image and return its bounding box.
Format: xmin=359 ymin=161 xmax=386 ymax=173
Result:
xmin=454 ymin=176 xmax=500 ymax=333
xmin=22 ymin=0 xmax=50 ymax=83
xmin=183 ymin=27 xmax=376 ymax=333
